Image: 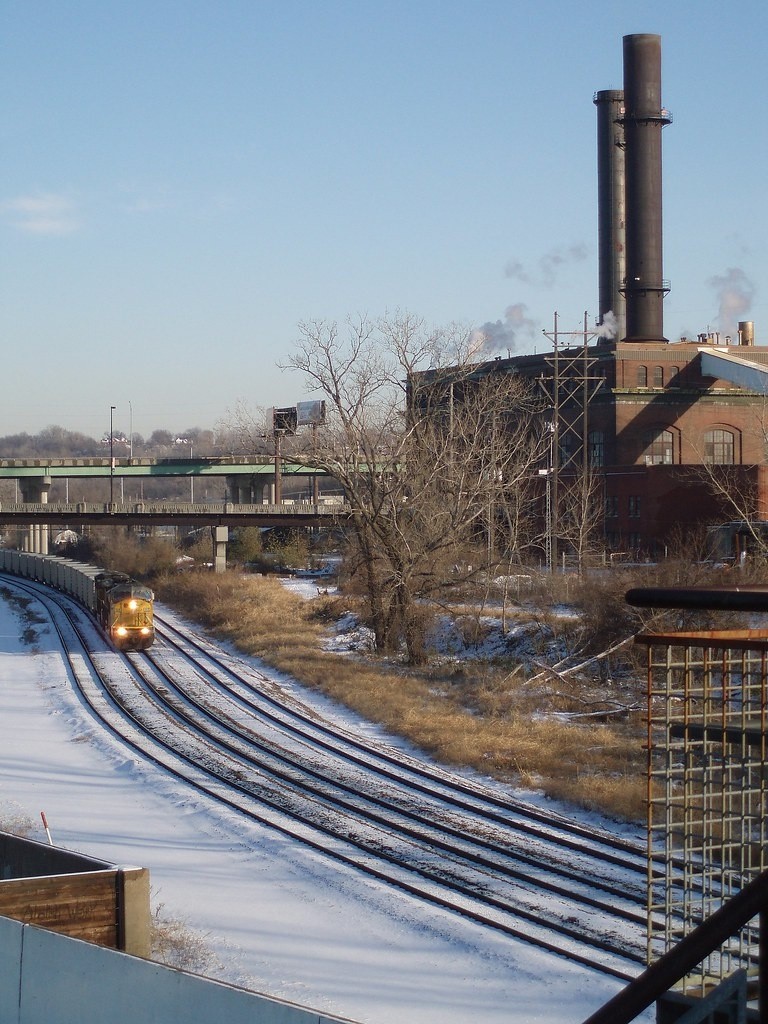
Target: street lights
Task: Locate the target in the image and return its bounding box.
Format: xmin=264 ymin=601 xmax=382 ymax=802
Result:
xmin=128 ymin=400 xmax=133 ymax=459
xmin=110 ymin=406 xmax=117 ymax=505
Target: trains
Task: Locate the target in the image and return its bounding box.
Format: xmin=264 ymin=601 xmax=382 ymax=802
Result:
xmin=0 ymin=548 xmax=155 ymax=652
